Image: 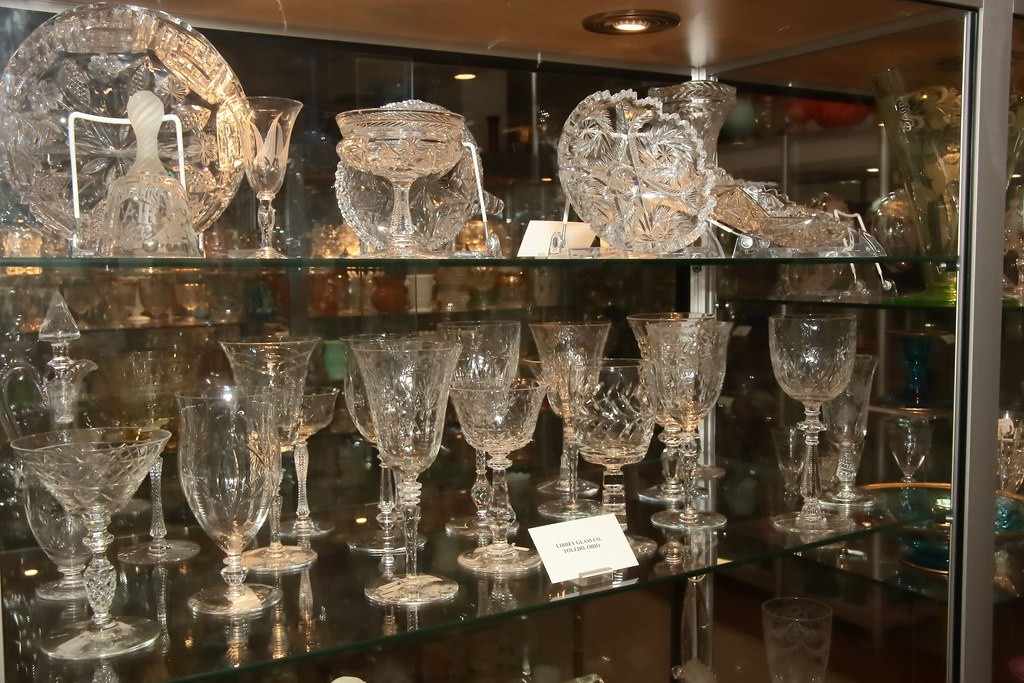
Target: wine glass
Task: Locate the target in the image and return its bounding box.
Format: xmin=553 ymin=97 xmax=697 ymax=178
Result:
xmin=335 ymin=110 xmax=468 ymax=258
xmin=81 ymin=347 xmax=202 ymax=565
xmin=768 ymin=313 xmax=858 ymax=534
xmin=225 ymin=96 xmax=304 ymax=259
xmin=626 ymin=313 xmax=733 ymax=532
xmin=349 ymin=340 xmax=463 ymax=607
xmin=888 ymin=412 xmax=931 ymax=514
xmin=448 ymin=377 xmax=551 ymax=576
xmin=522 ymin=322 xmax=613 ymax=521
xmin=568 ymin=357 xmax=657 ymax=557
xmin=435 ymin=320 xmax=522 ymax=544
xmin=174 ymin=333 xmax=340 ymax=616
xmin=816 ymin=354 xmax=882 ymax=508
xmin=10 ymin=425 xmax=172 ymax=661
xmin=337 ymin=333 xmax=428 ymax=554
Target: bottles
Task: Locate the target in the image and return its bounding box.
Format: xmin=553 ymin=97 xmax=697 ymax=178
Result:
xmin=647 ymin=81 xmax=737 ymax=259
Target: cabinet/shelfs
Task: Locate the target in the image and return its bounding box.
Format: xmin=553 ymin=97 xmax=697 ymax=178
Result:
xmin=0 ymin=0 xmax=1024 ymax=683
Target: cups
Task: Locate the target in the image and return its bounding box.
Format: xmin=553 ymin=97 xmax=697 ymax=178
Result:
xmin=763 ymin=596 xmax=833 ymax=683
xmin=91 ymin=176 xmax=204 ymax=259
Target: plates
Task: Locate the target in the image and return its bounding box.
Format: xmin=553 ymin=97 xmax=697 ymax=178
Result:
xmin=707 ymin=164 xmax=847 ymax=250
xmin=557 ymin=89 xmax=715 ymax=252
xmin=0 ymin=3 xmax=255 ymax=253
xmin=326 ymin=99 xmax=486 ymax=251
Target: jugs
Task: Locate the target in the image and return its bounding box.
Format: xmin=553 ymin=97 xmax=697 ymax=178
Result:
xmin=0 ymin=288 xmax=99 ymax=600
